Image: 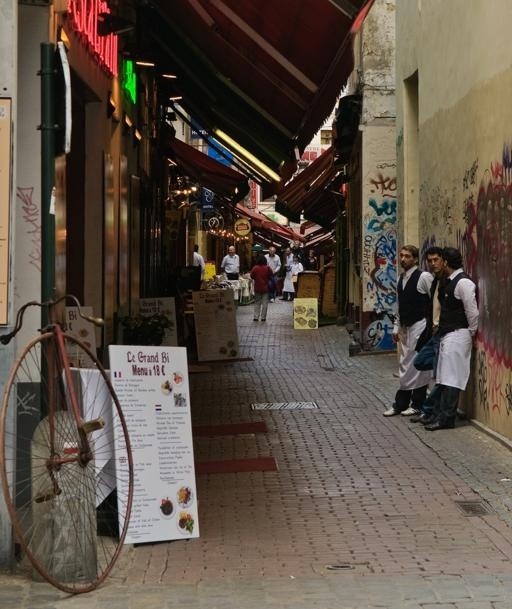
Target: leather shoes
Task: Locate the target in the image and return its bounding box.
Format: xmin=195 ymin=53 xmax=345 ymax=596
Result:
xmin=410 ymin=413 xmax=450 ymax=430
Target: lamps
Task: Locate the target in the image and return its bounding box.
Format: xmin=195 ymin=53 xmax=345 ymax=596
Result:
xmin=165 ymin=181 xmax=197 ymax=209
xmin=97 ymin=5 xmax=137 ymax=40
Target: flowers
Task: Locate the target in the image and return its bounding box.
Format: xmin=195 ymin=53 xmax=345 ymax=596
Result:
xmin=120 ymin=311 xmax=174 ymax=330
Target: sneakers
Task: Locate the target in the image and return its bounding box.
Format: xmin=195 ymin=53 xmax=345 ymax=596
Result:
xmin=400 ymin=407 xmax=421 ymax=415
xmin=382 ymin=408 xmax=399 ymax=416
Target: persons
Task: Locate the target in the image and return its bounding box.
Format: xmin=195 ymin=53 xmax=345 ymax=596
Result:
xmin=250 ymin=255 xmax=273 ymax=321
xmin=382 ymin=244 xmax=434 ymax=417
xmin=420 ymin=247 xmax=479 ymax=431
xmin=251 ymin=247 xmax=318 ymax=303
xmin=410 ymin=246 xmax=450 ymax=423
xmin=220 ymin=245 xmax=240 ymax=280
xmin=193 ymin=244 xmax=205 ymax=273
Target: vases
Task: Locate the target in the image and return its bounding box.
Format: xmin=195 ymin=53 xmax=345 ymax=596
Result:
xmin=124 ymin=332 xmax=164 ymax=345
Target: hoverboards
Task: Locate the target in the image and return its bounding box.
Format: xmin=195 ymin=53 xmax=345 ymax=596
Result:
xmin=0 ymin=290 xmax=136 ymax=595
